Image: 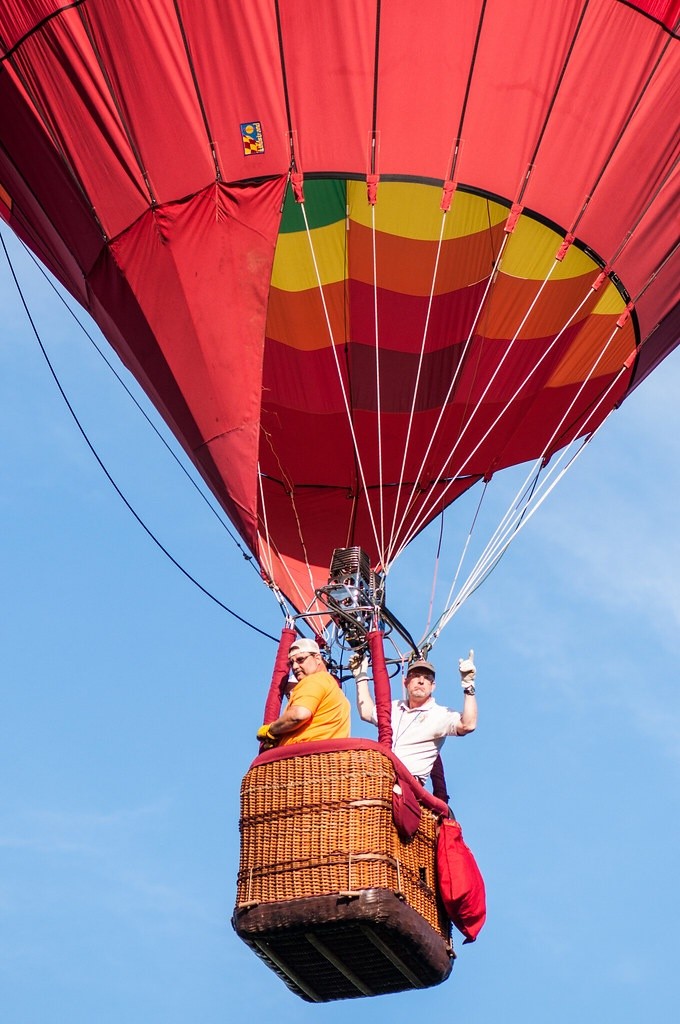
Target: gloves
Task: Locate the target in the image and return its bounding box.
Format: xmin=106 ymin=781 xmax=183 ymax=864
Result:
xmin=349 ymin=653 xmax=369 ymax=684
xmin=256 ymin=723 xmax=279 ymax=742
xmin=459 ymin=649 xmax=476 ymax=687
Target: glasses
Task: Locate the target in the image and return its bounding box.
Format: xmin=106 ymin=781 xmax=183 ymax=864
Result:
xmin=405 ymin=671 xmax=436 ymax=683
xmin=286 ymin=653 xmax=314 ymax=666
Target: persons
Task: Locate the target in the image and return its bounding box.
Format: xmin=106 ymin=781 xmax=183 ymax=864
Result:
xmin=257 ymin=639 xmax=351 ymax=747
xmin=348 ymin=649 xmax=477 ymax=786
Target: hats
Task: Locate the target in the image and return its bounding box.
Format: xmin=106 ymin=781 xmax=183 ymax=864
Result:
xmin=286 ymin=637 xmax=331 ymax=665
xmin=404 ymin=660 xmax=437 ymax=674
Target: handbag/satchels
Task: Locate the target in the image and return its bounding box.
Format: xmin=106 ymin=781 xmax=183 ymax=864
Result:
xmin=437 ymin=819 xmax=486 ymax=946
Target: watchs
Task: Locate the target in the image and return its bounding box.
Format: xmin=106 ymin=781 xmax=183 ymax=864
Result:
xmin=464 ymin=686 xmax=475 ymax=695
